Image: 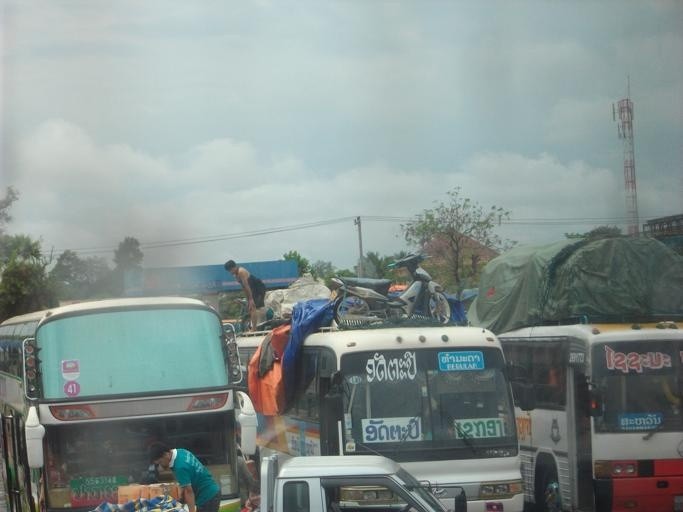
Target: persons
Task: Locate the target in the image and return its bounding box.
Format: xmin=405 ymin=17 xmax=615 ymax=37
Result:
xmin=224 ymin=260 xmax=266 ymax=332
xmin=149 ymin=441 xmax=223 ymax=512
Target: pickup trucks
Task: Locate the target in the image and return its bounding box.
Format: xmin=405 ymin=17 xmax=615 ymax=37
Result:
xmin=237 ymin=453 xmax=449 ymax=512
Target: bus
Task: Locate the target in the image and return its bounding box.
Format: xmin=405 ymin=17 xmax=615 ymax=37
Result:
xmin=229 ymin=325 xmax=525 ymax=512
xmin=496 ymin=314 xmax=683 ymax=512
xmin=0 ymin=295 xmax=259 ymax=512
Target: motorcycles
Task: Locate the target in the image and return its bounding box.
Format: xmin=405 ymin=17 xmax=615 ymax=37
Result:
xmin=332 ymin=251 xmax=465 ymax=329
